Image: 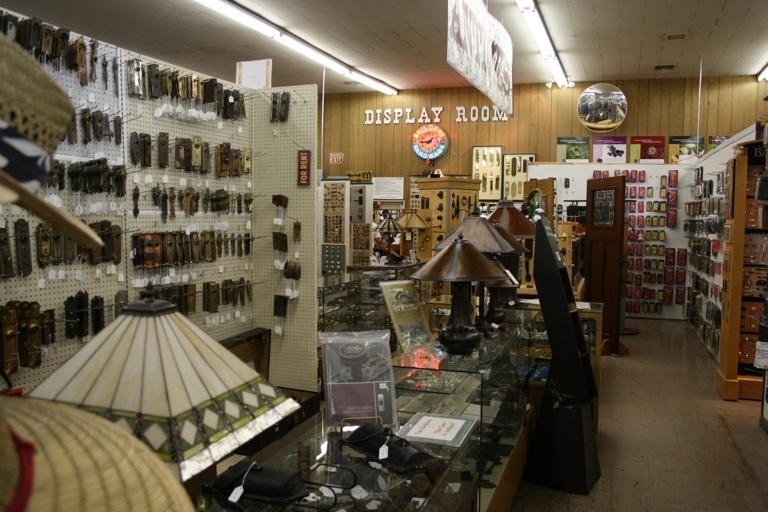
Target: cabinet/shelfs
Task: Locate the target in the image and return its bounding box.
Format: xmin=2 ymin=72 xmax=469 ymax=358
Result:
xmin=356 ymin=324 xmax=536 ymax=512
xmin=348 ymin=261 xmax=429 ymax=354
xmin=195 ymin=366 xmax=481 ymax=511
xmin=428 ymin=295 xmax=604 ymax=402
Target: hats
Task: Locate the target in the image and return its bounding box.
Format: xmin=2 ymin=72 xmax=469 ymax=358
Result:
xmin=0 ymin=34 xmax=108 ymax=252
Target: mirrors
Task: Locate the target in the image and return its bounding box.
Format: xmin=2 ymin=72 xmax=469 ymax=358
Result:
xmin=576 ymin=83 xmax=628 ymax=134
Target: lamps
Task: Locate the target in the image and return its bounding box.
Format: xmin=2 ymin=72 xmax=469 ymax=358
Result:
xmin=27 ymin=280 xmax=302 ymax=511
xmin=376 ymin=212 xmax=405 ymax=266
xmin=409 ymin=196 xmax=535 ymax=354
xmin=197 ymin=0 xmax=399 ymax=97
xmin=517 ymin=0 xmax=575 ymax=89
xmin=398 ymin=210 xmax=426 ymax=262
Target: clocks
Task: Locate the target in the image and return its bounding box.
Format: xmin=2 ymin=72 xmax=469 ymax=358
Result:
xmin=413 ymin=124 xmax=449 ymax=161
